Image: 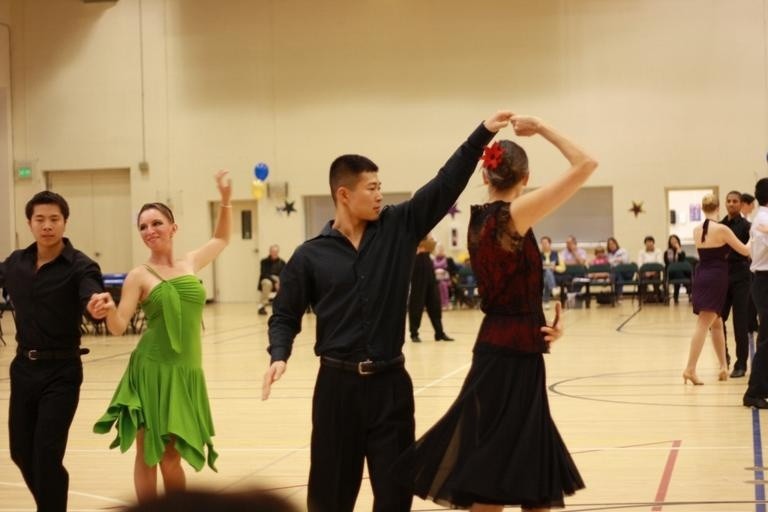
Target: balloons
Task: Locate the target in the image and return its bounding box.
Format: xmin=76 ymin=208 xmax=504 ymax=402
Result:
xmin=253 ymin=161 xmax=270 ymax=180
xmin=249 ymin=179 xmax=267 ymax=204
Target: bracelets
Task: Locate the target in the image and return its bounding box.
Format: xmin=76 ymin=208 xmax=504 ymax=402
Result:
xmin=219 ymin=199 xmax=235 ymax=212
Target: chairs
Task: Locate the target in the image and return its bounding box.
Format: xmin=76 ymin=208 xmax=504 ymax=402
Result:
xmin=447 ymin=258 xmax=696 ymax=306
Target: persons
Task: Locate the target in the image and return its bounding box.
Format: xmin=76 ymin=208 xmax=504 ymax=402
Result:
xmin=0 ymin=190 xmax=108 ymax=512
xmin=540 ymin=235 xmax=692 ymax=304
xmin=88 ymin=167 xmax=236 ymax=510
xmin=380 ymin=114 xmax=598 ymax=512
xmin=409 ymin=232 xmax=477 ymax=343
xmin=257 ymin=244 xmax=286 ymax=315
xmin=262 ymin=108 xmax=519 ymax=512
xmin=683 ymin=179 xmax=767 ymax=410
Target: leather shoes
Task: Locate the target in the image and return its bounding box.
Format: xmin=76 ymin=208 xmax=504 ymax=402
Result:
xmin=729 ymin=367 xmax=745 ymax=378
xmin=434 ymin=332 xmax=455 ymax=341
xmin=743 ymin=392 xmax=768 ymax=408
xmin=257 ymin=307 xmax=268 ymax=314
xmin=410 ymin=333 xmax=421 ymax=342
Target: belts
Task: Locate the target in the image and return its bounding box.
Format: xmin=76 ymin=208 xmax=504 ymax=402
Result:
xmin=320 ymin=354 xmax=405 ymax=375
xmin=17 ymin=347 xmax=91 ymax=361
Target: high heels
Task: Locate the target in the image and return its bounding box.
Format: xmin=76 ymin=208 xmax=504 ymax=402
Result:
xmin=718 ymin=368 xmax=727 ymax=381
xmin=683 ymin=371 xmax=705 ymax=386
